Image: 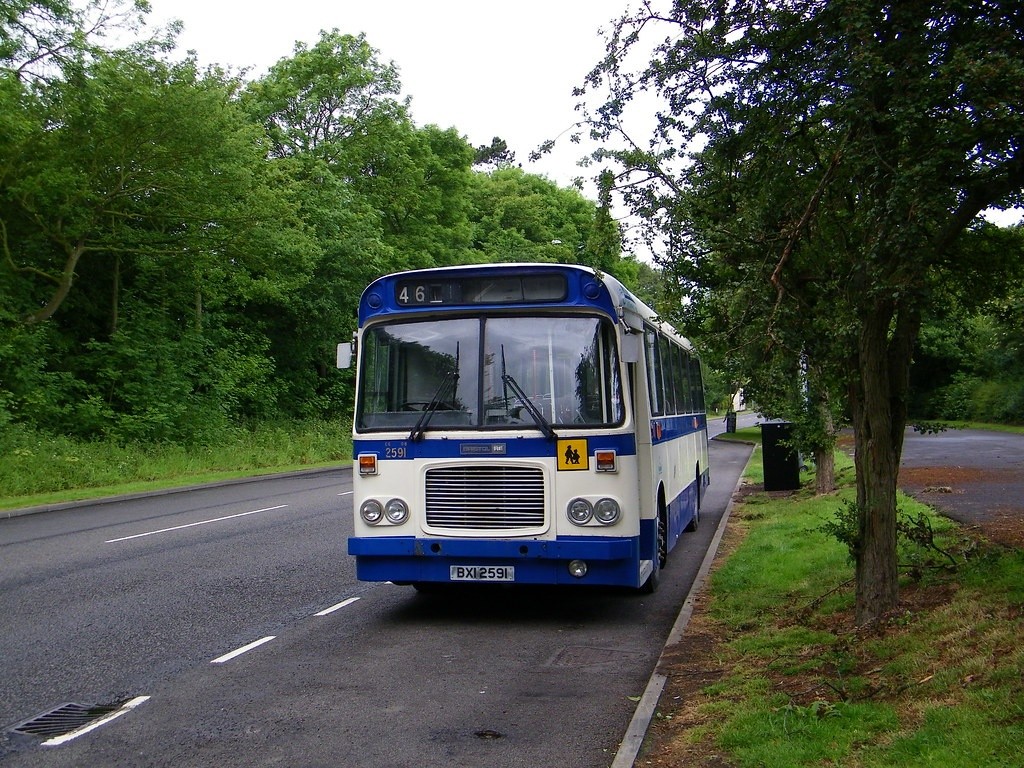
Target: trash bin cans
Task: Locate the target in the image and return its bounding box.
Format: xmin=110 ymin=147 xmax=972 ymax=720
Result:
xmin=759 ymin=419 xmax=801 ymax=491
xmin=726 ymin=412 xmax=737 ymax=433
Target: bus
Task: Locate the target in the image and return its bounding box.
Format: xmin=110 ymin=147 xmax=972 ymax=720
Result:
xmin=337 ymin=261 xmax=710 ymax=591
